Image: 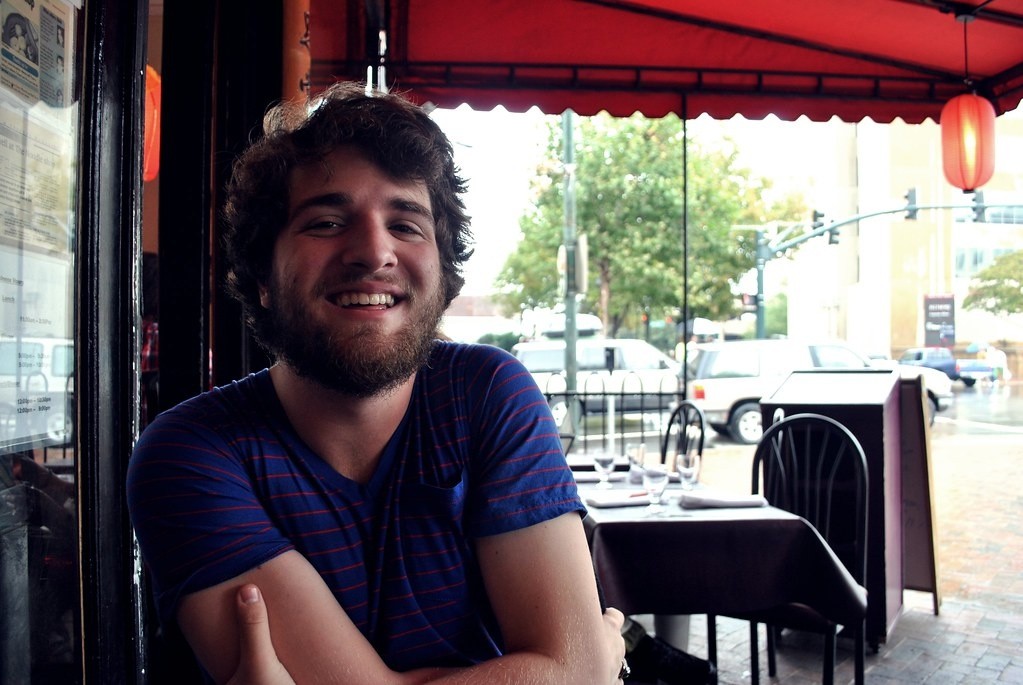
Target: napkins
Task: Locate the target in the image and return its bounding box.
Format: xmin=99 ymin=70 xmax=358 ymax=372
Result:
xmin=679 ymin=491 xmax=768 ymax=511
xmin=583 ymin=494 xmax=650 ymax=510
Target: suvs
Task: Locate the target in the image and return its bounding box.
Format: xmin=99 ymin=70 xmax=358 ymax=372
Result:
xmin=672 ymin=338 xmax=955 ymax=445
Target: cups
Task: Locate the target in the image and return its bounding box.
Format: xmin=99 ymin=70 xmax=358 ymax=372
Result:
xmin=676 ymin=454 xmax=701 ymax=495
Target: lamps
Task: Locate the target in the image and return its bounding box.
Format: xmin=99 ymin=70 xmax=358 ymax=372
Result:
xmin=939 ymin=7 xmax=998 ymax=193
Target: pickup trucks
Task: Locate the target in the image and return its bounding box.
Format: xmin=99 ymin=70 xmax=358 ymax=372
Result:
xmin=896 ymin=346 xmax=994 ymax=387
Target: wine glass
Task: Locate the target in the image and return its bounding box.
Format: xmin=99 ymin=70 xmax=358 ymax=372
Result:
xmin=642 ymin=463 xmax=670 ymax=516
xmin=594 ymin=452 xmax=615 ymax=488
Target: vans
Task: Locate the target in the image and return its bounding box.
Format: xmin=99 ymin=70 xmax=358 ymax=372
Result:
xmin=503 ymin=338 xmax=685 ymax=436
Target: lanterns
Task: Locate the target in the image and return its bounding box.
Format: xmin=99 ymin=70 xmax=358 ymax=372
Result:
xmin=941 ymin=92 xmax=996 ymax=195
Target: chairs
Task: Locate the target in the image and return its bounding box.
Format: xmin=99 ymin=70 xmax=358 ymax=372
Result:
xmin=655 ymin=400 xmax=707 ymax=485
xmin=706 ymin=412 xmax=870 ymax=685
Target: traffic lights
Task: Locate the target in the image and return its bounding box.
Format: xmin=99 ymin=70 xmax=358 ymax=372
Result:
xmin=971 ymin=191 xmax=985 ymax=223
xmin=904 ymin=187 xmax=917 ymax=220
xmin=741 ymin=293 xmax=758 ymax=312
xmin=811 ymin=210 xmax=825 ymax=236
xmin=828 ymin=219 xmax=839 ymax=244
xmin=642 ymin=306 xmax=649 ymax=325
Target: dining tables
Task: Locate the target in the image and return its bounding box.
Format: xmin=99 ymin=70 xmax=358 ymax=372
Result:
xmin=569 ymin=467 xmax=872 ymax=685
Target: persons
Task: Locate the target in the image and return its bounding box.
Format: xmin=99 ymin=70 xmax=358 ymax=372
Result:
xmin=122 ymin=84 xmax=627 ymax=685
